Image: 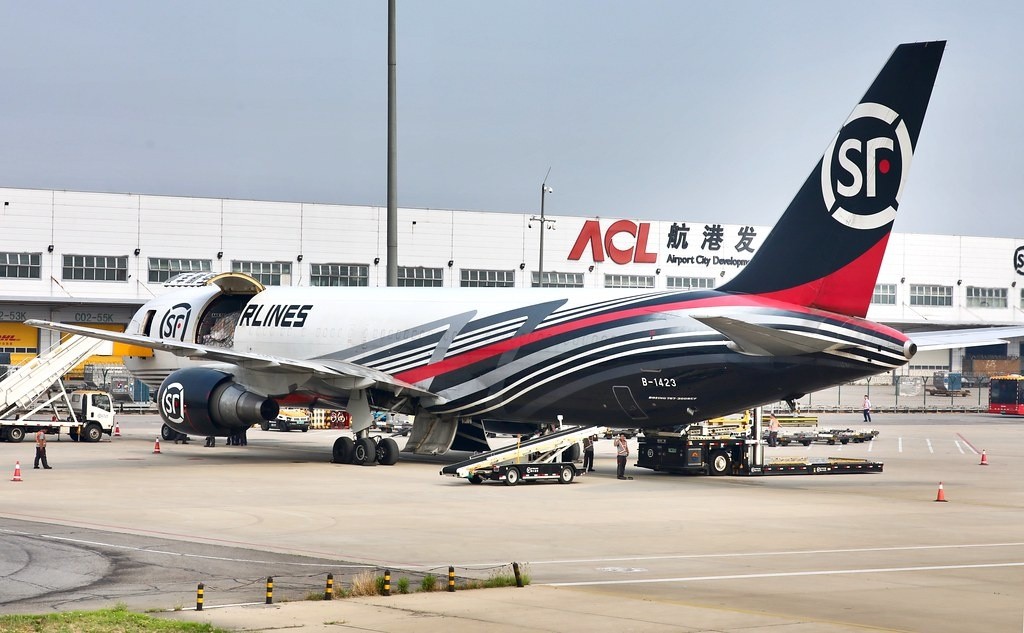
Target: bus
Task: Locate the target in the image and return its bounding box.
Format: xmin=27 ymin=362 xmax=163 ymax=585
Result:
xmin=988 ymin=374 xmax=1024 ymax=416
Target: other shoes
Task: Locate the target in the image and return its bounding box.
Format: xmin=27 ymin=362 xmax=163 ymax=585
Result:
xmin=204 ymin=444 xmax=215 ymax=447
xmin=33 ymin=467 xmax=40 ymax=469
xmin=174 ymin=438 xmax=189 ymax=444
xmin=44 ymin=466 xmax=52 ymax=469
xmin=588 ymin=468 xmax=595 ymax=472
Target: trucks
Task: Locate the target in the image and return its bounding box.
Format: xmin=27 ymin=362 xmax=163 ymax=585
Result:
xmin=0 ymin=332 xmax=116 ymax=443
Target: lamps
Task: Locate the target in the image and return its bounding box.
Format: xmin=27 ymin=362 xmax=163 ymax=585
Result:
xmin=448 ymin=261 xmax=453 ymax=267
xmin=298 ymin=255 xmax=303 ymax=261
xmin=589 ymin=266 xmax=594 ymax=271
xmin=218 ymin=252 xmax=223 ymax=258
xmin=48 ymin=245 xmax=53 ymax=252
xmin=134 ymin=249 xmax=140 ymax=255
xmin=720 ymin=271 xmax=725 ymax=276
xmin=656 ymin=269 xmax=661 ymax=274
xmin=520 ymin=264 xmax=525 ymax=269
xmin=375 ymin=258 xmax=379 ymax=264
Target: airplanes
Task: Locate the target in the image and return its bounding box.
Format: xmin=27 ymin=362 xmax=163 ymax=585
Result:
xmin=21 ymin=37 xmax=950 ymax=466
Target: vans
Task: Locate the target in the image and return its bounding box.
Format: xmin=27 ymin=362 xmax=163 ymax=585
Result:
xmin=258 ymin=406 xmax=311 ymax=432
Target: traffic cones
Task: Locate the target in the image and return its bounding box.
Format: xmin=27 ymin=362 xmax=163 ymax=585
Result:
xmin=10 ymin=461 xmax=24 ymax=481
xmin=152 ymin=437 xmax=162 ymax=454
xmin=113 ymin=422 xmax=122 ymax=436
xmin=933 ymin=482 xmax=948 ymax=503
xmin=978 ymin=449 xmax=988 ymax=465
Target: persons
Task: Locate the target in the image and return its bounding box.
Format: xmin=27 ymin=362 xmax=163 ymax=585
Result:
xmin=174 ymin=433 xmax=189 ymax=444
xmin=614 ymin=433 xmax=629 ymax=478
xmin=864 ymin=395 xmax=872 ymax=422
xmin=204 ymin=436 xmax=216 ymax=447
xmin=583 ymin=436 xmax=595 ymax=471
xmin=769 ymin=414 xmax=782 ymax=447
xmin=793 ymin=410 xmax=798 ymax=422
xmin=33 ymin=426 xmax=52 ymax=469
